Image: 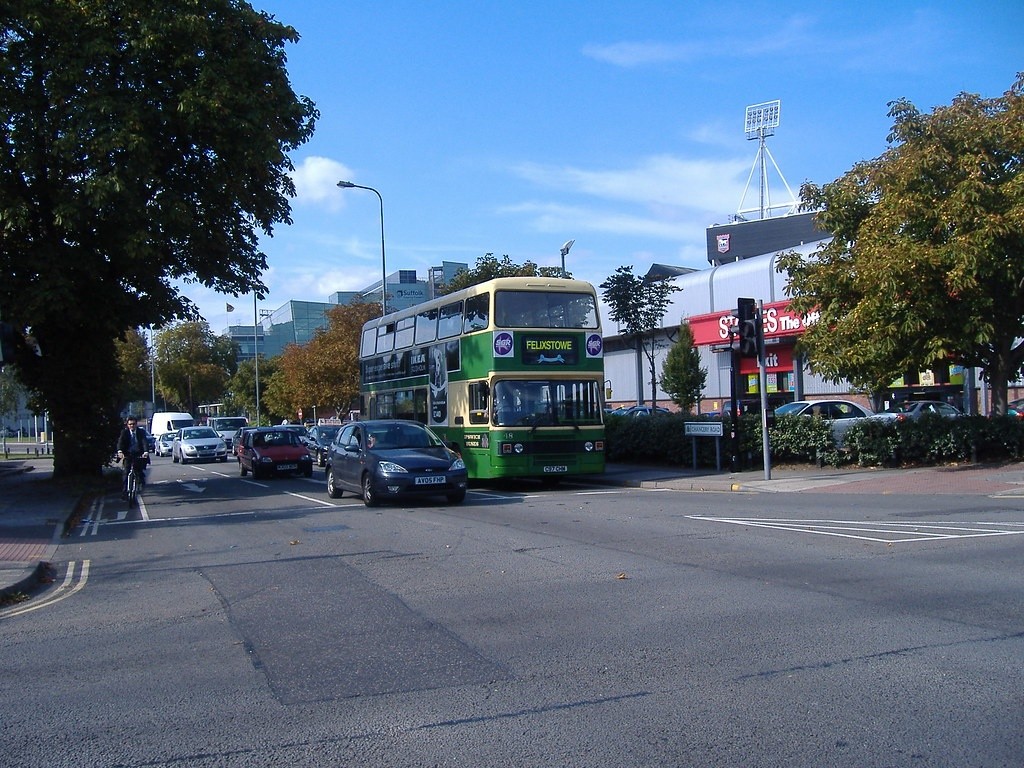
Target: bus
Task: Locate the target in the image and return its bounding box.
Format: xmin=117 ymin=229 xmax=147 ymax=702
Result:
xmin=359 ymin=277 xmax=614 ymax=488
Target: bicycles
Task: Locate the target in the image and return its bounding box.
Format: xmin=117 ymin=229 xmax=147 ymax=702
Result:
xmin=116 ymin=455 xmax=149 ymax=508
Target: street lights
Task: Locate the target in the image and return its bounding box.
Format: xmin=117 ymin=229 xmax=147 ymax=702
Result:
xmin=251 ymin=291 xmax=266 ymax=429
xmin=558 ymin=239 xmax=576 ymax=277
xmin=334 ymin=180 xmax=387 ymax=317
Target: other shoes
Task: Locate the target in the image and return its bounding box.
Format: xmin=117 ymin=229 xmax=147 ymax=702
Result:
xmin=122 ymin=492 xmax=128 ymax=500
xmin=138 ymin=483 xmax=143 ymax=492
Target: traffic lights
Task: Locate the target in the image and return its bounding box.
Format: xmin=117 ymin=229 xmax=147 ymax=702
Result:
xmin=730 ymin=298 xmax=757 ymax=359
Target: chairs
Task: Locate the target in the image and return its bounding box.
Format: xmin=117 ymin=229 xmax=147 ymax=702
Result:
xmin=806 ymin=405 xmax=829 ymax=419
xmin=385 ymin=430 xmax=410 ymax=445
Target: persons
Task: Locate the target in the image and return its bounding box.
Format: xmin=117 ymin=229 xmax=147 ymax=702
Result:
xmin=256 ymin=434 xmax=267 ymax=447
xmin=299 ymin=428 xmax=304 ymax=435
xmin=281 ymin=418 xmax=289 ymax=425
xmin=366 ymin=431 xmax=378 ymax=448
xmin=484 ymin=389 xmax=513 ymax=417
xmin=116 ymin=415 xmax=151 ymax=501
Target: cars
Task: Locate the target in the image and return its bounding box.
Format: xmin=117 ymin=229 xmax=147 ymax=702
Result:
xmin=770 ymin=399 xmax=893 ymax=450
xmin=989 ymin=397 xmax=1024 ymax=418
xmin=324 ymin=418 xmax=469 ymax=508
xmin=137 ymin=412 xmax=345 ymax=481
xmin=871 ymin=400 xmax=964 ymax=429
xmin=603 ymin=403 xmax=721 ymax=419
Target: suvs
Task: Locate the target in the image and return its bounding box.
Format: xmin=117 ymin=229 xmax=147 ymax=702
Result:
xmin=721 ymin=399 xmax=759 ymax=422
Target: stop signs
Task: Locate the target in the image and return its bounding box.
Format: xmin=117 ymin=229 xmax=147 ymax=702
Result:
xmin=298 ymin=408 xmax=302 ymax=419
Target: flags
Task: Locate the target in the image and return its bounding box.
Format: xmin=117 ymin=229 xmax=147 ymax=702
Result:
xmin=227 ymin=303 xmax=234 ymax=312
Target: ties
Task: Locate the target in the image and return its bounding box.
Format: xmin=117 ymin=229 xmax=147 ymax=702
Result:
xmin=132 ymin=433 xmax=135 ymax=443
